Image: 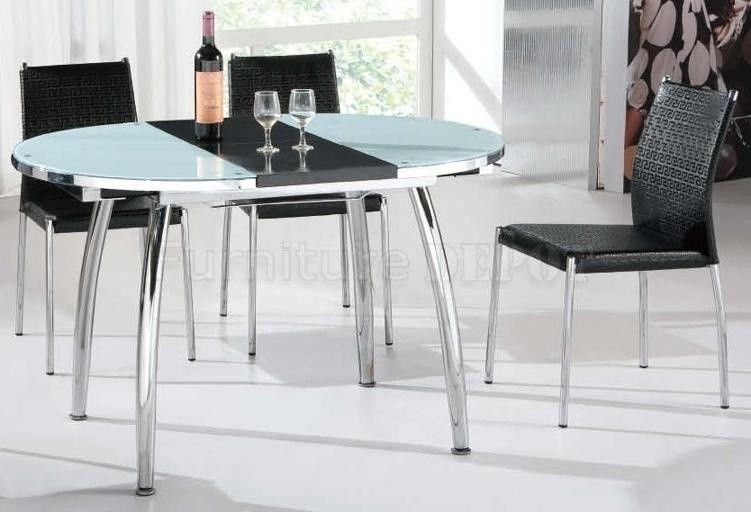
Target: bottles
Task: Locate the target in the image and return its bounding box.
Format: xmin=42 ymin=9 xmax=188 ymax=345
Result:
xmin=195 ymin=12 xmax=223 ymax=144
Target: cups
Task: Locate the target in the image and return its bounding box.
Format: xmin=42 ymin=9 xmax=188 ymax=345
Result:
xmin=260 ymin=152 xmax=276 ymax=173
xmin=289 ymin=89 xmax=316 ymax=151
xmin=294 ymin=149 xmax=313 ymax=173
xmin=254 ymin=91 xmax=282 ymax=153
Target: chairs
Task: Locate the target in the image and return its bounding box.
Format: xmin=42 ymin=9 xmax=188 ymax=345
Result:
xmin=15 ymin=58 xmax=198 ymax=375
xmin=221 ymin=49 xmax=394 ymax=358
xmin=485 ymin=76 xmax=740 ymax=428
